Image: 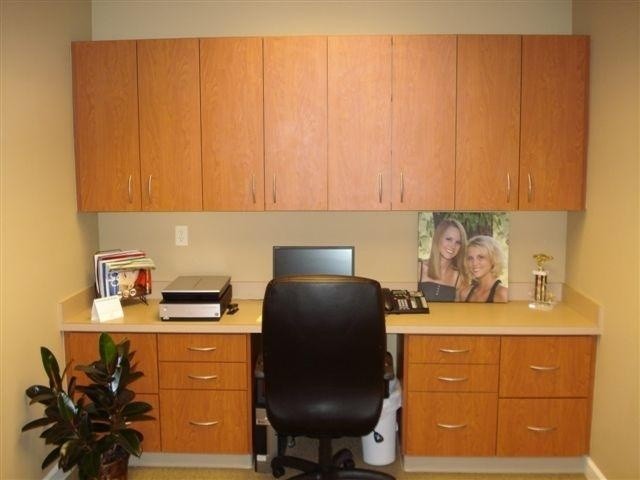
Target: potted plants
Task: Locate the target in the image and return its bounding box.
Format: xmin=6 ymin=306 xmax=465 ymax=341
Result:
xmin=20 ymin=333 xmax=156 ymax=480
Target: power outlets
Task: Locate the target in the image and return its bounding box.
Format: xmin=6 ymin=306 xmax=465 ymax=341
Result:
xmin=174 ymin=224 xmax=189 ymax=246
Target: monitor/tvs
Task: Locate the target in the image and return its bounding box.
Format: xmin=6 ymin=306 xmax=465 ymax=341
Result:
xmin=272 ymin=244 xmax=356 ymax=279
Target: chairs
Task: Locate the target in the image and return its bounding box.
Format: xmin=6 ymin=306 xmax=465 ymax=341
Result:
xmin=255 ymin=273 xmax=399 ymax=480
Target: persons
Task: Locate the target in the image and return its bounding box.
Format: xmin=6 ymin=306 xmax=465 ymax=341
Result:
xmin=461 ymin=236 xmax=509 ymax=304
xmin=417 ymin=219 xmax=469 ymax=302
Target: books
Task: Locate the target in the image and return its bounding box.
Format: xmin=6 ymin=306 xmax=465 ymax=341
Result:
xmin=94 ymin=249 xmax=156 ymax=299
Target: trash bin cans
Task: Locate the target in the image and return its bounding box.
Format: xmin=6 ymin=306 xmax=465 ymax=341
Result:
xmin=361 ymin=377 xmax=402 ymax=467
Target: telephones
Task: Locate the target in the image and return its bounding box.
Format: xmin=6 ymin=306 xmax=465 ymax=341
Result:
xmin=383 ymin=288 xmax=430 ymax=313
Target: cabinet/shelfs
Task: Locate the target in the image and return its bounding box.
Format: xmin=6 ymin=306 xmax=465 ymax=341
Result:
xmin=202 ymin=32 xmax=327 ymax=213
xmin=59 ymin=324 xmax=159 ymax=467
xmin=495 ymin=334 xmax=598 ymax=472
xmin=454 ymin=33 xmax=591 ymax=213
xmin=396 ymin=334 xmax=500 ymax=474
xmin=326 ymin=34 xmax=458 ymax=210
xmin=157 ymin=331 xmax=255 ymax=469
xmin=70 ymin=37 xmax=202 ymax=214
